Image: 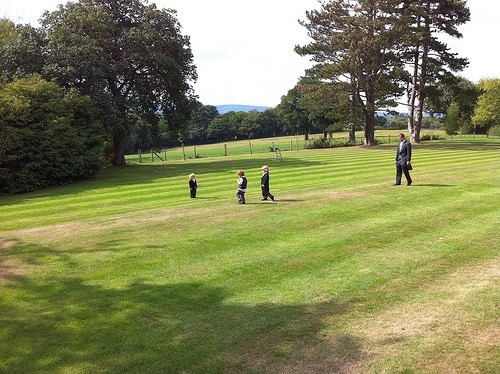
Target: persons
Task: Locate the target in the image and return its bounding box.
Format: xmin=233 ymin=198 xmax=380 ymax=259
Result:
xmin=260 ymin=165 xmax=275 ymax=201
xmin=236 ymin=170 xmax=248 ymax=203
xmin=393 ymin=134 xmax=412 ymax=186
xmin=189 ymin=173 xmax=197 ymax=198
xmin=349 ymin=127 xmax=352 ymax=139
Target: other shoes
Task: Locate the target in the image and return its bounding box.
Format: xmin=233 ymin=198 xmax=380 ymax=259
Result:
xmin=272 ymin=196 xmax=274 ymax=201
xmin=394 ymin=184 xmax=400 ymax=186
xmin=407 ymin=181 xmax=412 ymax=185
xmin=262 ymin=198 xmax=267 ymax=201
